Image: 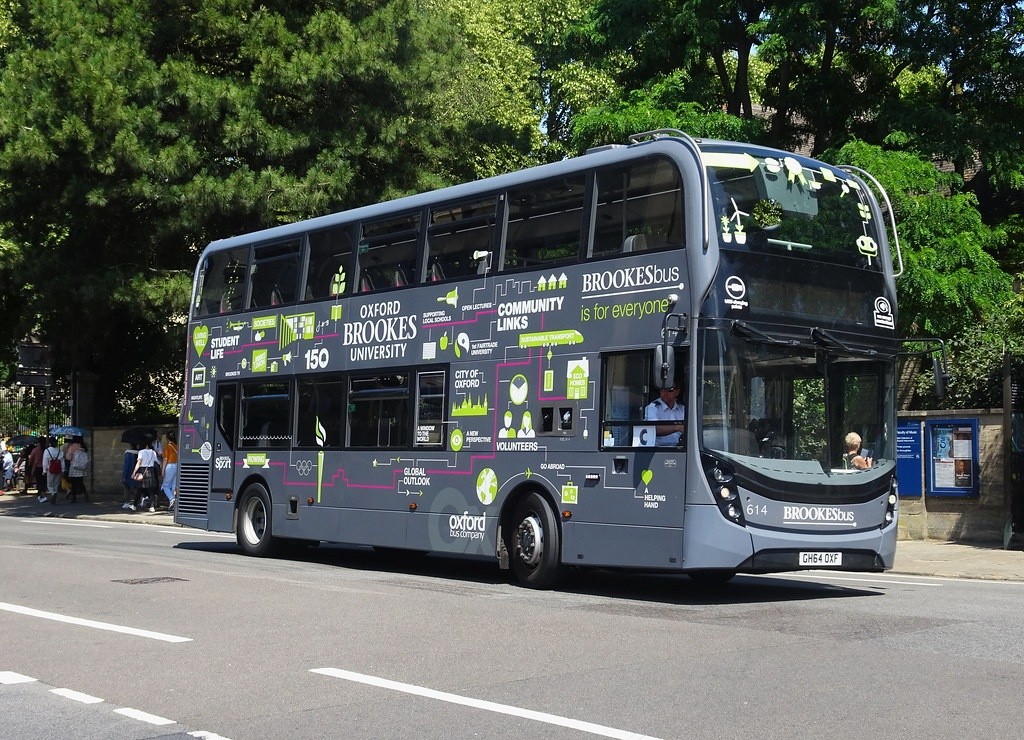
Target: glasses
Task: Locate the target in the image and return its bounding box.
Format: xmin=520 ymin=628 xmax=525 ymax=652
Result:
xmin=660 ymin=387 xmax=677 ymax=392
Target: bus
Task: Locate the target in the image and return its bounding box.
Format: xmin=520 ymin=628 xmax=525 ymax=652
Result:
xmin=174 ymin=129 xmax=951 ymax=590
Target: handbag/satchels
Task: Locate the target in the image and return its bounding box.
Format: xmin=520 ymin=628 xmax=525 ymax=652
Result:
xmin=134 ymin=473 xmax=143 ymax=481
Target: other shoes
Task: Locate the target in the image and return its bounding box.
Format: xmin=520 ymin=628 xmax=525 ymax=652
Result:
xmin=128 ymin=504 xmax=137 ymax=512
xmin=84 ymin=493 xmax=89 ymax=501
xmin=65 ymin=491 xmax=71 ymax=499
xmin=122 ymin=503 xmax=131 ymax=508
xmin=147 ymin=507 xmax=155 ymax=512
xmin=168 ymin=498 xmax=175 ymax=511
xmin=38 ymin=496 xmax=41 ymax=502
xmin=51 ymin=494 xmax=56 ymax=504
xmin=40 ymin=497 xmax=48 ymax=503
xmin=69 ymin=498 xmax=77 ymax=503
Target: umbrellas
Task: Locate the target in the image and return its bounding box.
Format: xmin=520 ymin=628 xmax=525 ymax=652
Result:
xmin=121 ymin=427 xmax=157 ymax=442
xmin=48 ymin=426 xmax=89 ymax=436
xmin=6 ymin=435 xmax=39 ymax=448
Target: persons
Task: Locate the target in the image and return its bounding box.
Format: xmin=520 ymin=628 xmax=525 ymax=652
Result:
xmin=42 ymin=436 xmax=65 ymax=504
xmin=122 ymin=436 xmax=163 ymax=512
xmin=0 ymin=437 xmax=37 ymax=494
xmin=645 ymin=381 xmax=684 ymax=445
xmin=65 ymin=436 xmax=90 ymax=503
xmin=29 ymin=436 xmax=48 ymax=503
xmin=956 ymin=461 xmax=965 ymax=475
xmin=845 ymin=432 xmax=872 ymax=469
xmin=60 ymin=440 xmax=71 ymax=498
xmin=162 ymin=431 xmax=177 ymax=510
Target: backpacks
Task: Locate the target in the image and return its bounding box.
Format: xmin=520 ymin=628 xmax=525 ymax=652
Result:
xmin=47 ymin=448 xmax=62 ymax=475
xmin=67 ymin=447 xmax=88 ymax=470
